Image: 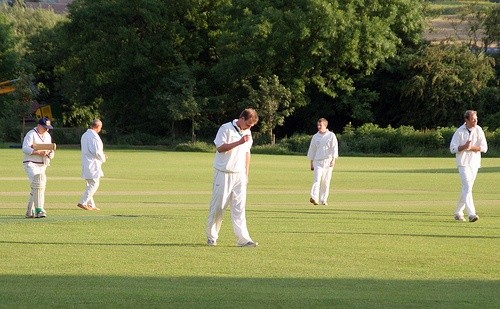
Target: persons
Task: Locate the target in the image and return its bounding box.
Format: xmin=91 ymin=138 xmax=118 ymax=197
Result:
xmin=450 ymin=110 xmax=488 ymax=222
xmin=307 ymin=118 xmax=338 ymax=205
xmin=22 ymin=118 xmax=55 ymax=219
xmin=77 ymin=119 xmax=108 ymax=211
xmin=206 ymin=108 xmax=258 ymax=248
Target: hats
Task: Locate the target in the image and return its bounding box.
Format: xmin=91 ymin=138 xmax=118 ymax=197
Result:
xmin=37 ymin=117 xmax=54 ymax=129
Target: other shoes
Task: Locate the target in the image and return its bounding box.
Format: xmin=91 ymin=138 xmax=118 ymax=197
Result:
xmin=246 ymin=241 xmax=258 ymax=247
xmin=207 ymin=239 xmax=218 ymax=246
xmin=468 ymin=215 xmax=479 ymax=222
xmin=92 ymin=207 xmax=100 ymax=211
xmin=25 ymin=212 xmax=36 ymax=218
xmin=322 ymin=201 xmax=327 ymax=205
xmin=78 ymin=203 xmax=91 ymax=210
xmin=36 ymin=212 xmax=47 ymax=218
xmin=310 ymin=197 xmax=318 ymax=206
xmin=455 ymin=215 xmax=465 ymax=221
